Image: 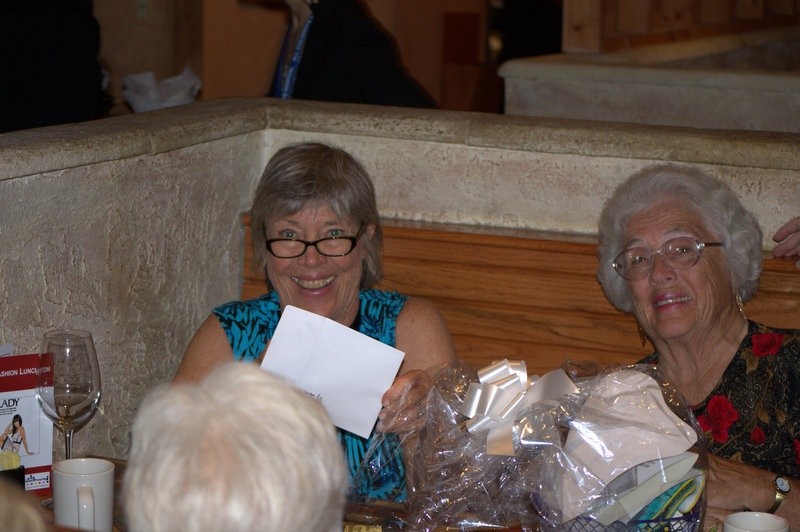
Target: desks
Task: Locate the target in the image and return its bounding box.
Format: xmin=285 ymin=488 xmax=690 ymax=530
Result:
xmin=45 ymin=454 xmax=414 ymax=532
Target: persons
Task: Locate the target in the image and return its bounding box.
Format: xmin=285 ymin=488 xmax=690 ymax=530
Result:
xmin=122 ymin=359 xmax=346 ymax=532
xmin=0 ymin=414 xmax=34 ymax=456
xmin=172 ymin=142 xmax=459 ymax=502
xmin=596 ymin=162 xmax=800 ymax=532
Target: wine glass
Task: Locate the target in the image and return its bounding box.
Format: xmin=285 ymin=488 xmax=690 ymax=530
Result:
xmin=35 ymin=330 xmax=98 ymax=459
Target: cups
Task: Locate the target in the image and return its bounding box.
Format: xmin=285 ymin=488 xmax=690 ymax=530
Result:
xmin=722 ymin=512 xmax=789 ymax=532
xmin=51 ymin=459 xmax=114 ymax=532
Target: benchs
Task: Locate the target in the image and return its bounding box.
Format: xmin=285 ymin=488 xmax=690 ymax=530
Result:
xmin=240 ymin=211 xmax=800 ymax=376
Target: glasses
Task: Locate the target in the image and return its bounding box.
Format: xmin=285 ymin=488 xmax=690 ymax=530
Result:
xmin=263 ymin=223 xmax=367 ymax=258
xmin=613 ymin=237 xmax=726 ymax=281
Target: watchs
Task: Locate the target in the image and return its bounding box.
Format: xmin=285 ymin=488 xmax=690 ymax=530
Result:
xmin=768 ymin=473 xmax=791 ymax=514
